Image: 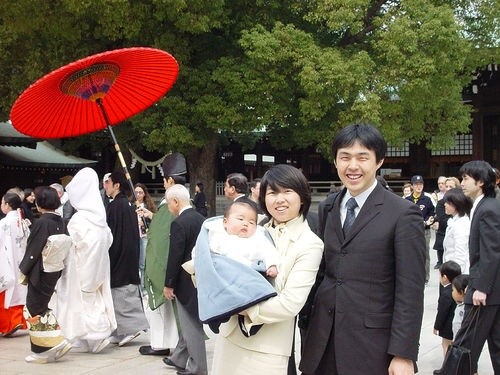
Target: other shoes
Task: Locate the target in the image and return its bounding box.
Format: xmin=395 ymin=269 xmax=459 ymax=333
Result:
xmin=176 ymin=369 xmax=192 ymax=375
xmin=139 ymin=345 xmax=170 ymax=355
xmin=163 ymin=357 xmax=176 ymax=366
xmin=434 ymin=264 xmax=440 ymax=269
xmin=433 ymin=370 xmax=441 ymax=375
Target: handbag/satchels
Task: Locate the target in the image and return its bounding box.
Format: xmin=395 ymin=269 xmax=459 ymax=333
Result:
xmin=442 ymin=342 xmax=474 ymax=375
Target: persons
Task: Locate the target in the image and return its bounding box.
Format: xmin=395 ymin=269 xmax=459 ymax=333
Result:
xmin=402 ymin=183 xmax=412 ymax=199
xmin=138 ymin=153 xmax=187 ymax=355
xmin=0 ymin=167 xmax=155 ymax=364
xmin=224 ymin=173 xmax=263 ymax=214
xmin=431 ymin=177 xmax=462 ymax=268
xmin=191 ymin=183 xmax=208 ymax=217
xmin=452 ymin=274 xmax=469 ymax=341
xmin=181 ymin=164 xmax=325 ymax=375
xmin=432 ymin=176 xmax=447 ymax=206
xmin=434 ymin=261 xmax=461 ymax=355
xmin=433 ymin=160 xmax=500 ymax=375
xmin=405 ymin=175 xmax=435 ymax=287
xmin=164 ymin=184 xmax=207 ymax=375
xmin=298 ymin=124 xmax=427 ymax=375
xmin=492 ymin=168 xmax=500 ymax=198
xmin=443 ymin=187 xmax=471 ymax=275
xmin=195 ymin=201 xmax=280 ymax=324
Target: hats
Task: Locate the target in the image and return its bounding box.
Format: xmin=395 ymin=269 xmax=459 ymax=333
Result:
xmin=159 ymin=153 xmax=187 ymax=178
xmin=408 ymin=175 xmax=424 ymax=183
xmin=103 ymin=173 xmax=112 ymax=182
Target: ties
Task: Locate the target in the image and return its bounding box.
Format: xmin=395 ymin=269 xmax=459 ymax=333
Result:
xmin=342 ymin=198 xmax=358 ymax=240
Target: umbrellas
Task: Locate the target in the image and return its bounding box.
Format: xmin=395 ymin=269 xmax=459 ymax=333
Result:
xmin=10 ymin=46 xmax=180 ymax=232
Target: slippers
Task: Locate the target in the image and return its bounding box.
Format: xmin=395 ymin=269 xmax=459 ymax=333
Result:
xmin=118 ymin=332 xmax=140 ymax=347
xmin=68 ymin=345 xmax=89 ymax=353
xmin=110 ymin=336 xmax=122 ymax=343
xmin=25 ymin=353 xmax=48 ymax=363
xmin=55 ymin=342 xmax=72 ymax=359
xmin=92 ymin=338 xmax=110 ymax=353
